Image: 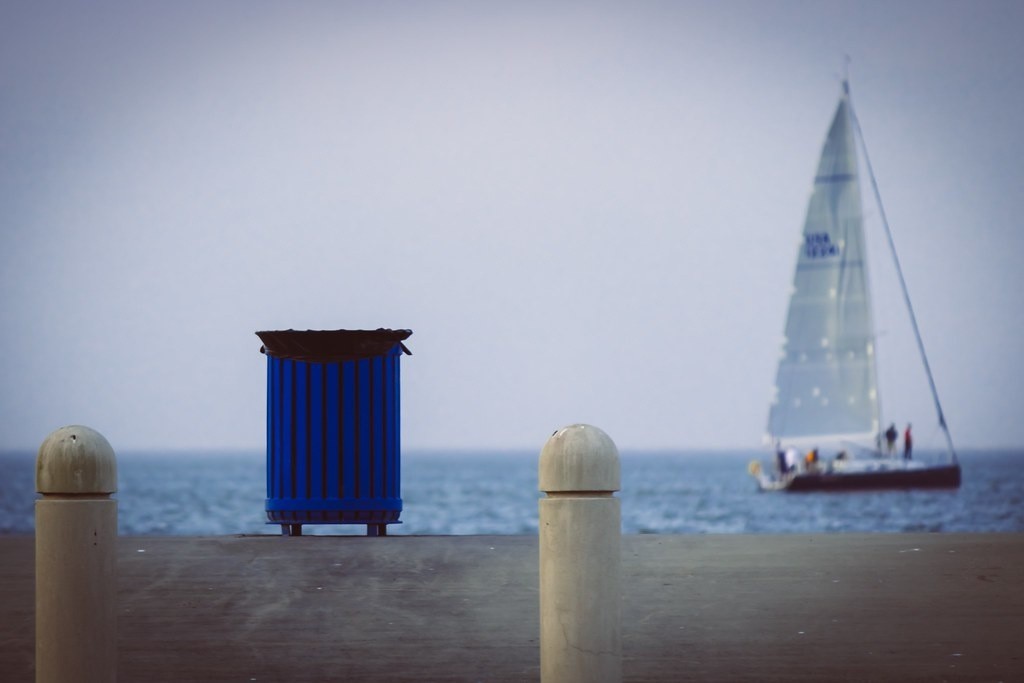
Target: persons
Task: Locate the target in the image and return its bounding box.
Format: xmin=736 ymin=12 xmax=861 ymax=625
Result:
xmin=886 ymin=423 xmax=898 ymax=459
xmin=904 ymin=423 xmax=913 ymax=460
xmin=778 ymin=444 xmax=818 ymax=483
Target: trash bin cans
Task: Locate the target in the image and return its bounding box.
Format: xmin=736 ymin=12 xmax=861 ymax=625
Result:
xmin=256 ymin=324 xmax=415 ymax=537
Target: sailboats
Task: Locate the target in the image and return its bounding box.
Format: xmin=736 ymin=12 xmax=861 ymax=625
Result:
xmin=746 ymin=57 xmax=963 ymax=495
